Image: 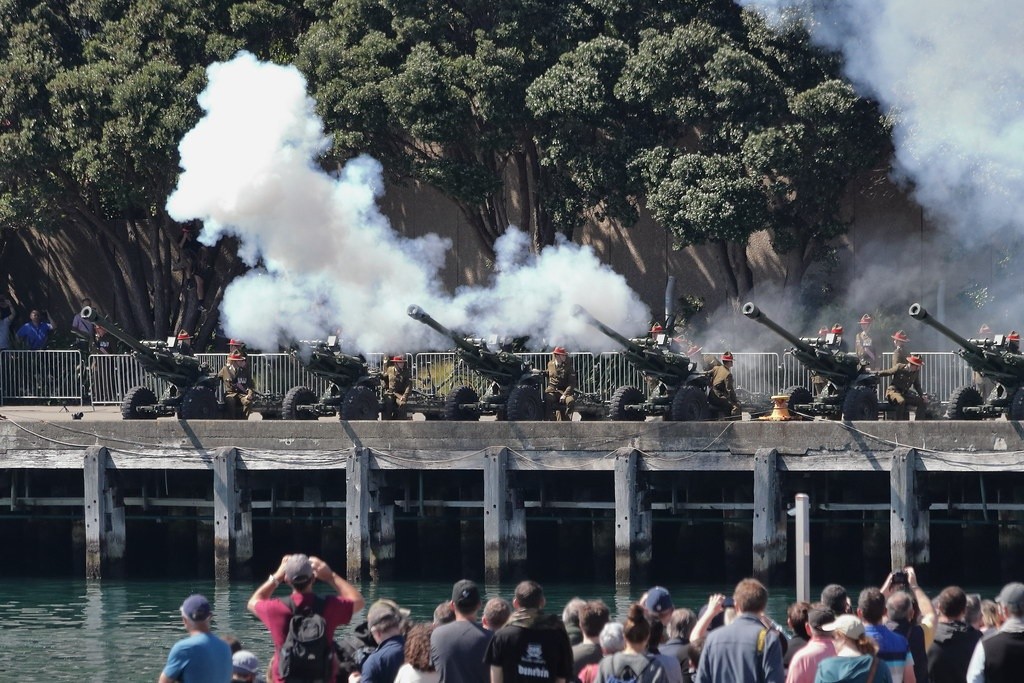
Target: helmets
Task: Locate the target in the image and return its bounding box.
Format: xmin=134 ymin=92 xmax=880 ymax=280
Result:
xmin=1008 ymin=331 xmax=1020 ymax=340
xmin=648 ymin=323 xmax=664 ymax=332
xmin=176 ymin=330 xmax=193 ymax=340
xmin=721 ymin=351 xmax=735 ymax=361
xmin=906 ymin=356 xmax=926 ymax=366
xmin=831 ymin=323 xmax=844 ymax=333
xmin=686 ymin=345 xmax=703 ymax=357
xmin=553 ymin=346 xmax=568 ymax=355
xmin=227 ymin=338 xmax=242 ymax=345
xmin=891 ymin=331 xmax=910 ymax=342
xmin=390 ymin=354 xmax=406 ymax=362
xmin=858 ymin=314 xmax=871 ymax=323
xmin=229 ymin=350 xmax=245 ymax=361
xmin=979 ymin=323 xmax=992 ymax=334
xmin=816 ymin=325 xmax=831 ymax=334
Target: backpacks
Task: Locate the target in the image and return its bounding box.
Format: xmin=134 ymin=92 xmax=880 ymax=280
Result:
xmin=276 ymin=596 xmax=331 ymax=683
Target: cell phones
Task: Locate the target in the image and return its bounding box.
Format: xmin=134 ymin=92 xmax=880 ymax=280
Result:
xmin=721 ymin=597 xmax=735 ymax=607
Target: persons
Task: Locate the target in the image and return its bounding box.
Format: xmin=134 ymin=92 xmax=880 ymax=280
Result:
xmin=648 ymin=322 xmax=665 ymax=394
xmin=855 ymin=313 xmax=882 ymax=371
xmin=871 ymin=355 xmax=928 ymax=420
xmin=95 ymin=325 xmax=117 ymax=406
xmin=811 ymin=323 xmax=849 ymax=395
xmin=15 ymin=308 xmax=54 ymax=350
xmin=892 ymin=329 xmax=911 ymax=367
xmin=375 ymin=350 xmax=413 ymax=420
xmin=177 ymin=329 xmax=193 ymax=345
xmin=72 ymin=298 xmax=94 ymax=357
xmin=973 ymin=323 xmax=1020 ymax=398
xmin=218 ymin=338 xmax=254 ymax=420
xmin=688 ymin=346 xmax=742 ymax=421
xmin=0 ymin=299 xmax=16 ymax=360
xmin=156 ymin=555 xmax=1024 ymax=683
xmin=545 ymin=346 xmax=575 ymax=421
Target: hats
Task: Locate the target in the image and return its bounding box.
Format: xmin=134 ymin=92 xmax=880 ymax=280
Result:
xmin=232 ymin=650 xmax=259 ymax=673
xmin=179 ymin=595 xmax=212 ymax=623
xmin=645 ymin=586 xmax=673 ymax=612
xmin=367 ymin=599 xmax=396 ymax=630
xmin=822 ymin=615 xmax=866 ymax=640
xmin=452 ymin=579 xmax=479 ymax=607
xmin=377 ymin=600 xmax=411 ymax=617
xmin=995 ymin=582 xmax=1024 ymax=605
xmin=285 ymin=554 xmax=313 ymax=584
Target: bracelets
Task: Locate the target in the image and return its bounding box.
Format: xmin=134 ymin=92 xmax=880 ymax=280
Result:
xmin=269 ymin=574 xmax=279 ymax=587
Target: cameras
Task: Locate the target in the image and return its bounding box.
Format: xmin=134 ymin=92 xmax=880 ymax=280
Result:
xmin=892 ymin=572 xmax=908 ymax=584
